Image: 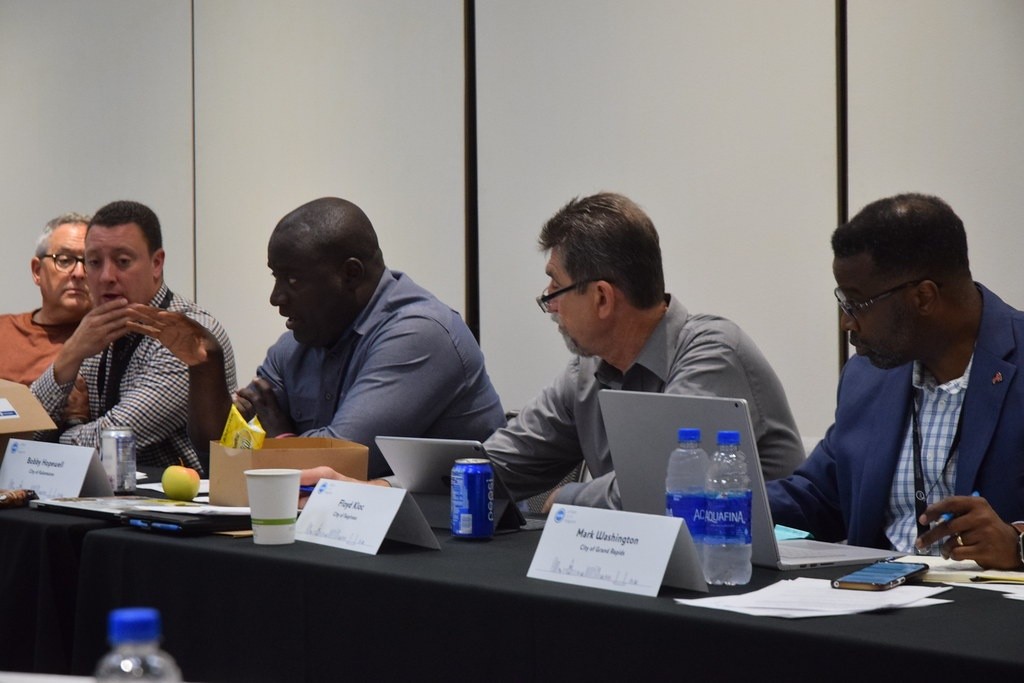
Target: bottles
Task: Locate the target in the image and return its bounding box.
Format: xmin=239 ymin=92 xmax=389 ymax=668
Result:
xmin=665 ymin=428 xmax=713 ymax=569
xmin=703 ymin=430 xmax=753 ymax=585
xmin=95 ymin=608 xmax=181 ymax=683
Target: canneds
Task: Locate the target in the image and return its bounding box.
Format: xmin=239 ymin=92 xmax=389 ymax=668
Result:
xmin=449 ymin=457 xmax=495 ymax=539
xmin=99 ymin=426 xmax=137 ymax=494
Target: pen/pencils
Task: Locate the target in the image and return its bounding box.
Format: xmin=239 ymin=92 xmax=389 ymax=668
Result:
xmin=300 ymin=486 xmax=316 ymax=491
xmin=936 ymin=491 xmax=981 ymax=525
xmin=128 ymin=519 xmax=181 ymax=530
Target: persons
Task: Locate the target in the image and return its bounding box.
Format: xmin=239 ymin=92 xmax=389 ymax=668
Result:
xmin=0 ymin=212 xmax=94 ymax=389
xmin=26 ymin=200 xmax=239 ymax=479
xmin=763 ymin=193 xmax=1023 ymax=571
xmin=299 ymin=192 xmax=806 ymax=513
xmin=124 ymin=197 xmax=508 ymax=481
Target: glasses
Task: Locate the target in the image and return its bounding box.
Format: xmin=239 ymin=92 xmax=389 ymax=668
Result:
xmin=834 ymin=281 xmax=921 ymax=323
xmin=536 ymin=281 xmax=578 ymax=314
xmin=37 ymin=253 xmax=88 ymax=273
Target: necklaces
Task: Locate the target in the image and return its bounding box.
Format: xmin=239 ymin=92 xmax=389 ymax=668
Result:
xmin=908 ymin=388 xmax=962 ymax=539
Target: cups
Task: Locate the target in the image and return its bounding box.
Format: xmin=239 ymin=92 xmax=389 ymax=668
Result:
xmin=244 ymin=468 xmax=301 ymax=544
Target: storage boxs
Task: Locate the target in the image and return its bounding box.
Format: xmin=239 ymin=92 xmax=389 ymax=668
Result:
xmin=209 ymin=439 xmax=369 ymax=508
xmin=1 ymin=375 xmax=57 ymax=449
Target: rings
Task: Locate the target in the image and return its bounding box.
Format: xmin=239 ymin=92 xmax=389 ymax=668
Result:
xmin=955 ymin=534 xmax=964 ymax=547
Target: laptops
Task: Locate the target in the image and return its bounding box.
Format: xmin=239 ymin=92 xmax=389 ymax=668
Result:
xmin=597 ymin=388 xmax=909 ymax=570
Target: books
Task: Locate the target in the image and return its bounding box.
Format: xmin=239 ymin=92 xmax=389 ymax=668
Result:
xmin=888 ymin=555 xmax=1024 ymax=586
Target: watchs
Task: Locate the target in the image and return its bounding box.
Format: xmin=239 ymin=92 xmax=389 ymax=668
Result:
xmin=1012 ymin=523 xmax=1024 ymax=564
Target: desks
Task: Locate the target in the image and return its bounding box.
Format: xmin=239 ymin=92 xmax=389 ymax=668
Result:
xmin=73 ymin=524 xmax=1024 ymax=683
xmin=0 ymin=505 xmax=112 ymax=672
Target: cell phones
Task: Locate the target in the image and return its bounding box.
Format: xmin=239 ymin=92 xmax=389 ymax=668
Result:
xmin=831 ymin=559 xmax=930 ymax=591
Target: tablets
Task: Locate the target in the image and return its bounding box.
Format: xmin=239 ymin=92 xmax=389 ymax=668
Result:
xmin=375 ymin=436 xmax=527 ymax=525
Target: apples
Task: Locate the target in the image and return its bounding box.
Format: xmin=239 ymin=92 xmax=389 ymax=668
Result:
xmin=161 ymin=457 xmax=201 ymax=501
